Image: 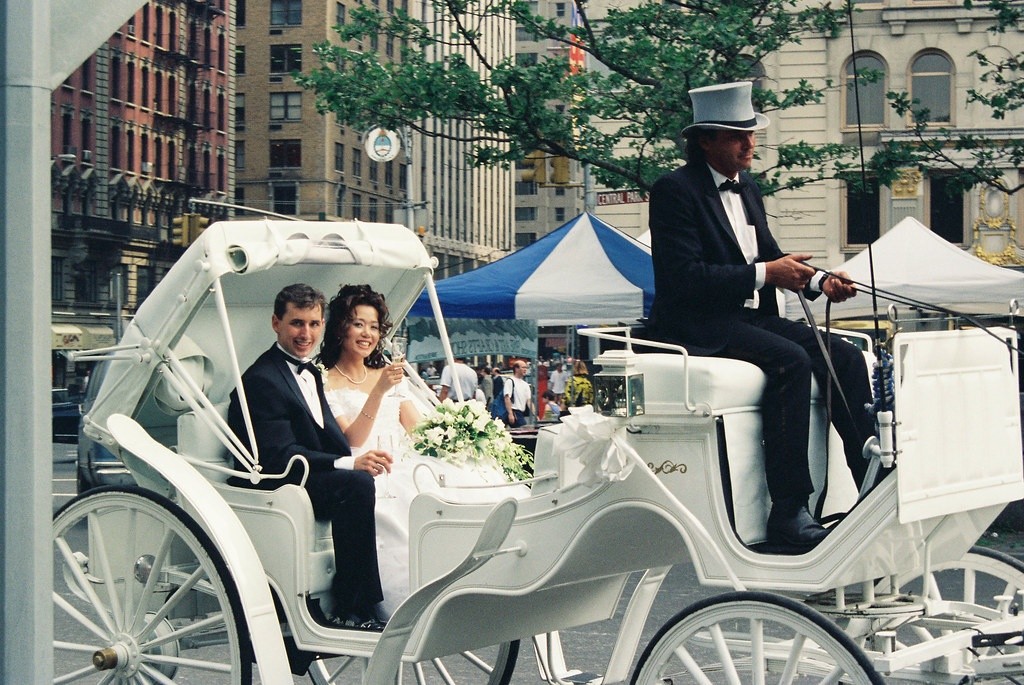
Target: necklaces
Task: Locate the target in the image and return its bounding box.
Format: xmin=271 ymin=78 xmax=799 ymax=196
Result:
xmin=335 ymin=364 xmax=368 ymax=384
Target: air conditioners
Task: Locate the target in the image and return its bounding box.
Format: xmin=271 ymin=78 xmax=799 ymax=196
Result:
xmin=84 ymin=150 xmax=91 ymax=160
xmin=128 ymin=25 xmax=135 ymax=34
xmin=141 ymin=162 xmax=152 ymax=172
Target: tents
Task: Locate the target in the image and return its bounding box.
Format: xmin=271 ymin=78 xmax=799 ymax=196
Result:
xmin=785 ymin=216 xmax=1024 ymax=324
xmin=406 ymin=211 xmax=654 ymax=320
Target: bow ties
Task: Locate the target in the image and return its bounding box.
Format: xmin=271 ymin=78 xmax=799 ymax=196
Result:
xmin=717 ymin=178 xmax=743 ymax=194
xmin=279 ymin=348 xmax=314 ymax=375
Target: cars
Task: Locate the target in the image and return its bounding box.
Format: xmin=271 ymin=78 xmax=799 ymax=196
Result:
xmin=52 ymin=388 xmax=83 ymax=440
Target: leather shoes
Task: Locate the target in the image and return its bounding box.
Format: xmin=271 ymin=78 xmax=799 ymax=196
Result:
xmin=766 ymin=502 xmax=832 ymax=547
xmin=329 ymin=603 xmax=384 ymax=630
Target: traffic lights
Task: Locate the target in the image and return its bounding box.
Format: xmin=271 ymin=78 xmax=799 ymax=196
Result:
xmin=521 ymin=143 xmax=546 ymax=184
xmin=549 ymin=137 xmax=571 ymax=185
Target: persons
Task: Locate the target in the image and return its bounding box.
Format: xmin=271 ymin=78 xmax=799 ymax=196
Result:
xmin=439 ymin=357 xmax=479 ymax=403
xmin=541 ymin=389 xmax=563 ymax=419
xmin=648 ymin=82 xmax=883 ymax=552
xmin=315 ymin=285 xmax=530 ymax=622
xmin=547 ymin=363 xmax=569 ymax=403
xmin=503 ymin=360 xmax=540 ymax=429
xmin=561 ymin=361 xmax=593 ymax=410
xmin=228 ymin=283 xmax=394 ymax=631
xmin=422 ymin=364 xmax=437 ymax=378
xmin=492 ymin=367 xmax=504 ymax=399
xmin=481 ymin=368 xmax=494 ymax=404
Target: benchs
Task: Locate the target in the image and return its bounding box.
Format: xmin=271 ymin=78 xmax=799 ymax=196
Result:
xmin=177 ymin=402 xmax=336 ymax=594
xmin=577 ymin=323 xmax=877 ymax=418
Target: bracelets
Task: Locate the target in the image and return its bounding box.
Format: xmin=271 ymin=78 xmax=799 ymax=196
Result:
xmin=819 ymin=273 xmax=830 ymax=291
xmin=362 ymin=410 xmax=375 ymax=420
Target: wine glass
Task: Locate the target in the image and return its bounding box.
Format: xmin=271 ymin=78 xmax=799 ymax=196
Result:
xmin=387 ymin=336 xmax=407 ymax=398
xmin=376 ymin=434 xmax=397 ymax=499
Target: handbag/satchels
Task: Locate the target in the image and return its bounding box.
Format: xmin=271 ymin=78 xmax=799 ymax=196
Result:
xmin=491 ymin=378 xmax=514 ymax=420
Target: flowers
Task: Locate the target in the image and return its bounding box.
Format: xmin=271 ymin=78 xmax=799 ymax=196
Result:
xmin=403 ymin=397 xmax=535 ymax=486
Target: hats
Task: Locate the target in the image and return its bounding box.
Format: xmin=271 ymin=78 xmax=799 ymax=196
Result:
xmin=680 ymin=81 xmax=770 ymax=134
